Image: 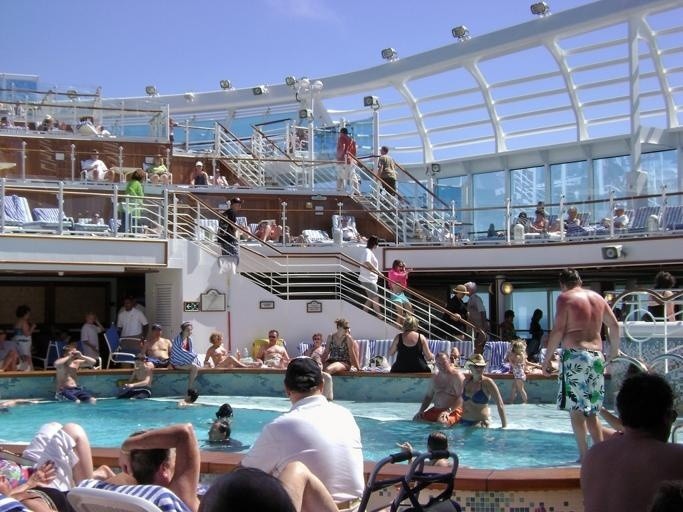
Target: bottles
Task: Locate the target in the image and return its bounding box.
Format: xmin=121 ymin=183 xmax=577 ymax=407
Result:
xmin=242 ymin=348 xmax=248 ymax=358
xmin=234 ymin=348 xmax=239 ymax=361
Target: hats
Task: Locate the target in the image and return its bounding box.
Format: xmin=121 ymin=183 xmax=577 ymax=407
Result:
xmin=231 ymin=197 xmax=244 ymax=204
xmin=452 ymin=285 xmax=469 ymax=294
xmin=467 ymin=353 xmax=487 ymax=366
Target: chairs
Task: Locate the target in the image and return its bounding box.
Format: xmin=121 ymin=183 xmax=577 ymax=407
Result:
xmin=390 ymin=450 xmax=462 ymax=512
xmin=32 ymin=322 xmax=561 ymax=373
xmin=1 ymin=195 xmax=121 ymax=232
xmin=81 ymin=161 xmax=112 ymax=182
xmin=357 ymin=452 xmax=425 ymax=512
xmin=478 ymin=206 xmax=683 ymax=240
xmin=67 ymin=479 xmax=193 ymax=512
xmin=195 ymin=215 xmax=356 ymax=246
xmin=144 ymin=156 xmax=172 ymax=182
xmin=79 ymin=124 xmax=99 ymax=136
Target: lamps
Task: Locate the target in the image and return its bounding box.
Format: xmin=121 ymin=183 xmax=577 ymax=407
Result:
xmin=381 ymin=48 xmax=399 ymax=62
xmin=286 ymin=76 xmax=323 ymax=119
xmin=220 ymin=80 xmax=234 ymax=90
xmin=531 ymin=2 xmax=550 ymax=17
xmin=252 ymin=85 xmax=269 ymax=96
xmin=452 ymin=26 xmax=471 ymax=42
xmin=428 ymin=163 xmax=442 ymax=176
xmin=67 ymin=90 xmax=79 ymax=101
xmin=146 ymin=86 xmax=158 ymax=96
xmin=602 ymin=245 xmax=625 ymax=260
xmin=364 ymin=96 xmax=380 ymax=110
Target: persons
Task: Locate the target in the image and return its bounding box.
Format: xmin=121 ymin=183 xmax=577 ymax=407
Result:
xmin=168 ymin=118 xmax=178 ymax=148
xmin=360 ymin=236 xmax=383 ymax=320
xmin=510 ymin=201 xmax=629 ymax=237
xmin=120 ymin=169 xmax=146 ymax=233
xmin=375 ymin=147 xmax=396 ymax=196
xmin=82 ymin=149 xmax=115 ymax=182
xmin=217 ymin=197 xmax=242 ymax=264
xmin=189 ymin=160 xmax=239 ymax=188
xmin=1 ymin=114 xmax=102 ymax=135
xmin=88 ymin=212 xmax=100 ymax=224
xmin=336 ymin=127 xmax=360 ymax=195
xmin=147 ymin=154 xmax=171 ymax=184
xmin=339 ymin=217 xmax=361 ymax=241
xmin=235 ymin=221 xmax=304 ymax=244
xmin=1 ymin=259 xmax=683 ymax=512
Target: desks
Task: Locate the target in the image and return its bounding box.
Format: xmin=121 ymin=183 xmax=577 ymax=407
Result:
xmin=110 ymin=167 xmax=142 ymax=183
